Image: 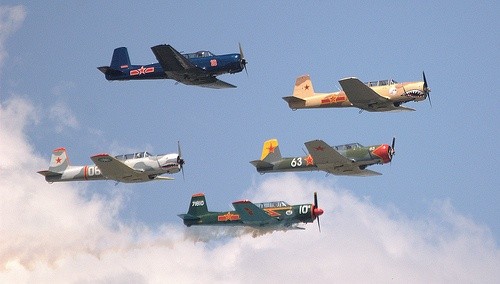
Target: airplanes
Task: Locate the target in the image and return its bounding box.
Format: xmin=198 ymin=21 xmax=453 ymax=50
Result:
xmin=281 ymin=71 xmax=431 ymax=113
xmin=39 ymin=141 xmax=184 ymax=184
xmin=177 ymin=193 xmax=324 ymax=232
xmin=249 ymin=138 xmax=396 ymax=177
xmin=98 ymin=42 xmax=249 ymax=92
